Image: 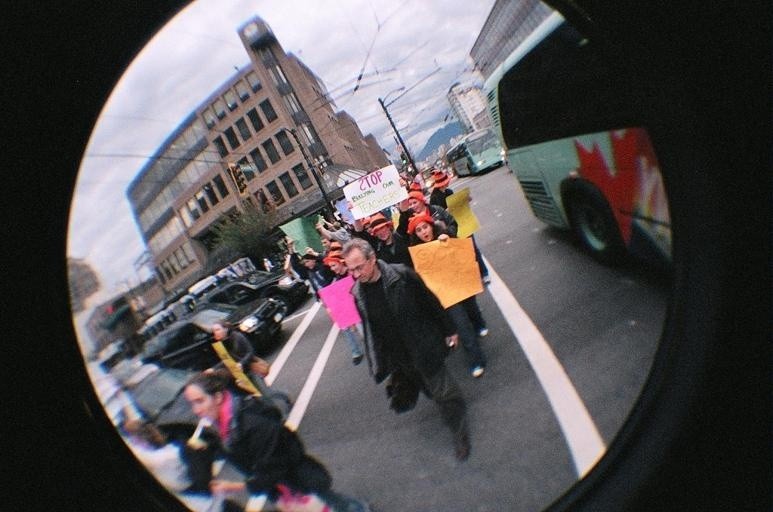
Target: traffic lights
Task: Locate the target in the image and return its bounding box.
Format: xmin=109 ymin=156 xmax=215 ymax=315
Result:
xmin=399 ymin=152 xmax=408 ymax=166
xmin=405 ymin=165 xmax=414 ymax=174
xmin=227 ymin=161 xmax=247 ymax=196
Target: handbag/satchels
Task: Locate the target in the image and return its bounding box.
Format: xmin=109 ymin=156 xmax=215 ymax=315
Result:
xmin=248 ymin=355 xmax=269 ymax=376
xmin=273 ymin=494 xmax=331 ymax=512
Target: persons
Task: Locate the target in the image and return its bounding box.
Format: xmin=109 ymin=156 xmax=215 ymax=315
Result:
xmin=201 ymin=321 xmax=294 ymax=425
xmin=281 ymin=162 xmax=488 ymax=378
xmin=178 ymin=364 xmax=380 ymax=512
xmin=341 ymin=237 xmax=471 ymax=461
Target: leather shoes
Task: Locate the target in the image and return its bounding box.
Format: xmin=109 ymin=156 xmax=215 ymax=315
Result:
xmin=351 ymin=354 xmax=363 ymax=366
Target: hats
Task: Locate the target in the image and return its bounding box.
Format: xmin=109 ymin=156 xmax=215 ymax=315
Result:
xmin=433 ymin=170 xmax=450 ymax=188
xmin=321 ymin=241 xmax=346 ymax=266
xmin=368 ymin=213 xmax=391 ymax=234
xmin=110 ymin=359 xmax=137 ymax=386
xmin=406 ymin=208 xmax=433 ymax=235
xmin=406 ymin=174 xmax=426 ymax=202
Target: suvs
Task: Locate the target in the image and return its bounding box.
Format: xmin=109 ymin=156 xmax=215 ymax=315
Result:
xmin=102 ymin=264 xmax=309 ymax=446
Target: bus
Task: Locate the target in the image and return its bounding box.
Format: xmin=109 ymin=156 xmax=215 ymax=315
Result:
xmin=444 ymin=127 xmax=503 ymax=179
xmin=187 ymin=254 xmax=256 ymax=303
xmin=477 ymin=11 xmax=674 ymax=279
xmin=95 ymin=336 xmax=137 ymax=374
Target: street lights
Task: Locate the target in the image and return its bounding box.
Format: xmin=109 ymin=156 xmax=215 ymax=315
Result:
xmin=381 ymin=85 xmax=419 ymax=175
xmin=278 ymin=125 xmax=343 ymax=226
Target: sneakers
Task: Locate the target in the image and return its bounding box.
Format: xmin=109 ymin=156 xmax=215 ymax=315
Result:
xmin=328 ymin=490 xmax=369 ymax=512
xmin=373 ymin=368 xmax=391 ymax=384
xmin=176 ymin=484 xmax=213 ymax=499
xmin=448 ymin=335 xmax=458 ymax=347
xmin=453 ymin=427 xmax=470 ymax=461
xmin=477 ymin=328 xmax=489 ymax=337
xmin=482 ymin=274 xmax=490 ymax=284
xmin=286 ymin=391 xmax=294 ymax=404
xmin=468 ymin=364 xmax=484 ymax=377
